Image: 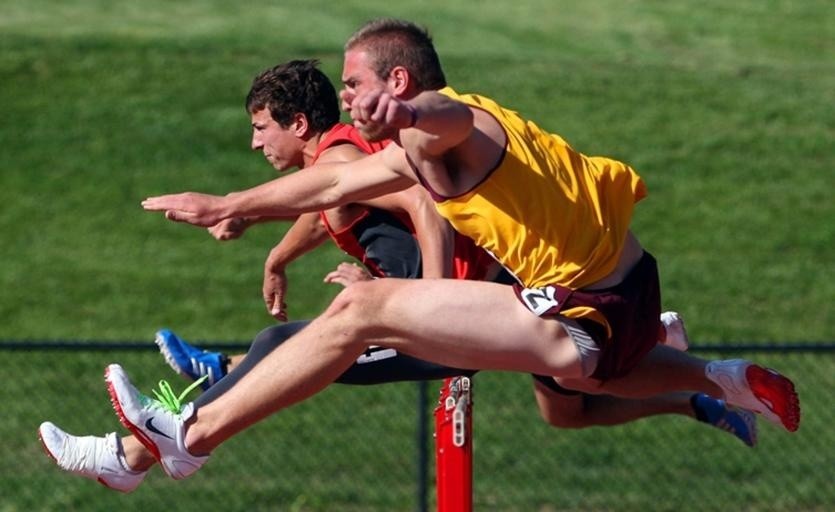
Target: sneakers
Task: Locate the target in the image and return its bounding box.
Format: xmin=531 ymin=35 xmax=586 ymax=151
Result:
xmin=39 ymin=421 xmax=146 ymax=494
xmin=661 ymin=310 xmax=688 ymax=351
xmin=697 ymin=395 xmax=758 ymax=447
xmin=704 ymin=357 xmax=800 ymax=432
xmin=154 ymin=328 xmax=226 ymax=392
xmin=102 ymin=363 xmax=211 ymax=480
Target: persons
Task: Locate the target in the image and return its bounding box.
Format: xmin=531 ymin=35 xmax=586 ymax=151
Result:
xmin=153 ymin=326 xmax=757 ymax=451
xmin=103 ymin=19 xmax=801 ymax=482
xmin=36 ymin=58 xmax=691 ymax=496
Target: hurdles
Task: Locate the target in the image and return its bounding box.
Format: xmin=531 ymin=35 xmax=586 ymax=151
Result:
xmin=434 ymin=375 xmax=473 ymax=512
